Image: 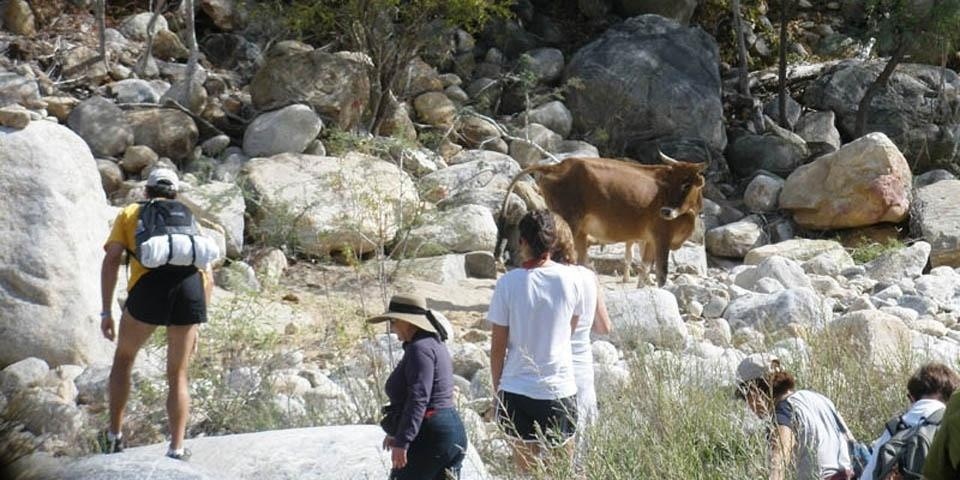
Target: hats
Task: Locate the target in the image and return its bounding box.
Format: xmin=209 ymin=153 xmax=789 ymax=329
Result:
xmin=365 ymin=294 xmax=454 ymax=344
xmin=146 ymin=168 xmax=180 ymax=191
xmin=733 ymin=352 xmax=785 ymax=396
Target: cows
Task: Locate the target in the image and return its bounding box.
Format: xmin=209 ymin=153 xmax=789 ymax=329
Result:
xmin=495 ymin=207 xmax=578 ymax=269
xmin=493 ymin=138 xmax=714 ymax=290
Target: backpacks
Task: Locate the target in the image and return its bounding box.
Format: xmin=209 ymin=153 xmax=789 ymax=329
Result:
xmin=125 ymin=196 xmax=202 ymax=271
xmin=870 ymin=409 xmax=945 ymax=480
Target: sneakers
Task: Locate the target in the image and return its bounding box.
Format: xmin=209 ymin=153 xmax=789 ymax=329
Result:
xmin=97 ymin=428 xmax=125 ymax=454
xmin=167 ymin=447 xmax=192 ymax=462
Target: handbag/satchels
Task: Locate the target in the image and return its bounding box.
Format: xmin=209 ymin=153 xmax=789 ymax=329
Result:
xmin=846 ymin=438 xmax=872 ymax=479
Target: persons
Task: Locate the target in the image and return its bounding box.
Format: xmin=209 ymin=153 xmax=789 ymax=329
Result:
xmin=867 ymin=360 xmax=959 ymax=479
xmin=97 ymin=176 xmax=215 ymax=465
xmin=736 ymin=351 xmax=860 ymax=480
xmin=365 ymin=292 xmax=468 ymax=480
xmin=550 ymin=210 xmax=613 ymax=479
xmin=484 ymin=210 xmax=583 ymax=479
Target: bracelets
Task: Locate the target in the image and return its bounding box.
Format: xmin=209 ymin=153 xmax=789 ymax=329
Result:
xmin=100 ymin=309 xmax=112 ymax=320
